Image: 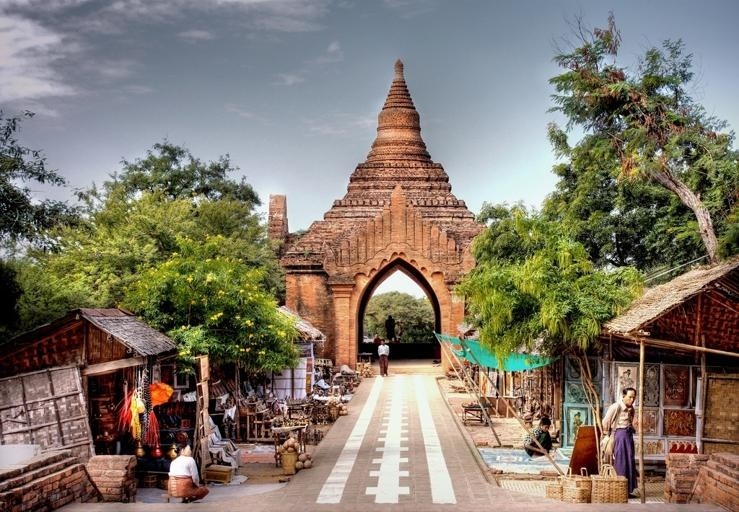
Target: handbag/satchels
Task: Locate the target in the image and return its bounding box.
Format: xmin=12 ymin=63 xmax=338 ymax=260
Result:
xmin=605 ymin=434 xmax=614 ymax=457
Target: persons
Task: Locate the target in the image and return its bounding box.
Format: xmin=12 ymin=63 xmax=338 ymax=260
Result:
xmin=602 ymin=389 xmax=640 ymax=499
xmin=377 ymin=339 xmax=391 ymax=377
xmin=524 ymin=415 xmax=553 ymax=458
xmin=572 ymin=410 xmax=583 ymax=439
xmin=374 ymin=334 xmax=381 ymax=345
xmin=385 ymin=315 xmax=398 ymax=344
xmin=167 ymin=442 xmax=210 ymax=503
xmin=614 ymin=365 xmax=697 ymax=455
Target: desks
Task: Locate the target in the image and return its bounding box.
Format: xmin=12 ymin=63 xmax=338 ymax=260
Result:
xmin=270 ymin=426 xmax=306 ymax=467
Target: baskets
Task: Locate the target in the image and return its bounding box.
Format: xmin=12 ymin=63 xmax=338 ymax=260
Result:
xmin=544 ymin=483 xmax=561 ymax=500
xmin=590 ymin=463 xmax=629 ymax=504
xmin=561 ymin=465 xmax=589 ymax=504
xmin=279 ymin=452 xmax=297 ymax=476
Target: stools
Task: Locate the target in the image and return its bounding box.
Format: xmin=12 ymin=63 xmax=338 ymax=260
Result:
xmin=461 ymin=401 xmax=492 ymax=426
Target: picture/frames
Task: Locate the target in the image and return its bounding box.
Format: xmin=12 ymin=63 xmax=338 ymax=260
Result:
xmin=563 ymin=402 xmax=592 ymax=449
